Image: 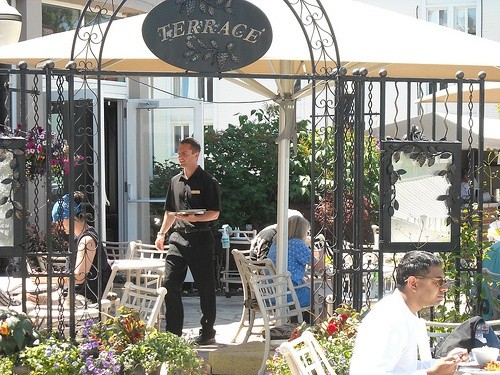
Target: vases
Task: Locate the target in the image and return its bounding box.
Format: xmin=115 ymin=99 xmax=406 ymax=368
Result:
xmin=125 ymin=359 xmax=145 ymax=375
xmin=11 ymin=364 xmax=31 ymax=375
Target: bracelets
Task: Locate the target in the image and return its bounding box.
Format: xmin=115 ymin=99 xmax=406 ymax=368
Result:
xmin=158 ymin=232 xmax=165 ymax=236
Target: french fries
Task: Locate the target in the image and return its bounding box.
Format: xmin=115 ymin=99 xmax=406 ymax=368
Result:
xmin=487 ymin=360 xmax=500 ymax=371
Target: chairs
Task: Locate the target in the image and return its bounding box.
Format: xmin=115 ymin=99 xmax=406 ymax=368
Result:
xmin=24 ymin=230 xmax=337 ymax=375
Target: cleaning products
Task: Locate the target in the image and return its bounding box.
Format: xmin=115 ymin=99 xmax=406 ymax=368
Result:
xmin=221 ymin=224 xmax=230 ymax=248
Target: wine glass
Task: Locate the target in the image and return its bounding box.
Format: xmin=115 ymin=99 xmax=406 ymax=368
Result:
xmin=245 ymin=225 xmax=252 ymax=237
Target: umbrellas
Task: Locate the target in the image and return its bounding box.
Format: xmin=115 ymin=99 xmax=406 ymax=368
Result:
xmin=0 ymin=0 xmax=500 ymax=339
xmin=417 ymin=82 xmax=500 ymax=102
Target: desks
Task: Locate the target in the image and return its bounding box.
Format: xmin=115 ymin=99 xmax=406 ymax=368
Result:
xmin=454 ymin=360 xmax=500 ymax=375
xmin=108 ymin=258 xmax=166 ymax=307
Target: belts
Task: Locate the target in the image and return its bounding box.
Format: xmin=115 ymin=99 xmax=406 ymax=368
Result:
xmin=173 ymin=227 xmax=213 ymax=233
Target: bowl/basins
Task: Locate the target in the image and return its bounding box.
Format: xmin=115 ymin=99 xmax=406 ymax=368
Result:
xmin=472 ymin=347 xmax=499 ymax=369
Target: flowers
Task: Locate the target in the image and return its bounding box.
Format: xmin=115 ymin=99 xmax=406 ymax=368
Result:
xmin=0 ymin=306 xmax=207 ymax=375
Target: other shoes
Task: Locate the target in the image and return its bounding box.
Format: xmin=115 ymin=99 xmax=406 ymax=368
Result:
xmin=191 ymin=334 xmax=216 ymax=346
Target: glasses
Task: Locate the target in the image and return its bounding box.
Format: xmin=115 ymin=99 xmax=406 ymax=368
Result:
xmin=404 ymin=275 xmax=446 ymax=286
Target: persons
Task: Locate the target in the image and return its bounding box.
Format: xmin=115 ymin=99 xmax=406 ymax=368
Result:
xmin=155 ymin=137 xmax=222 ymax=345
xmin=471 ymin=241 xmax=500 ymax=331
xmin=350 ymin=250 xmax=471 ymax=375
xmin=250 ymin=208 xmax=304 ymax=275
xmin=260 ymin=214 xmax=327 ymax=326
xmin=33 ymin=190 xmax=113 ymax=305
xmin=461 ymin=173 xmax=479 ymax=202
